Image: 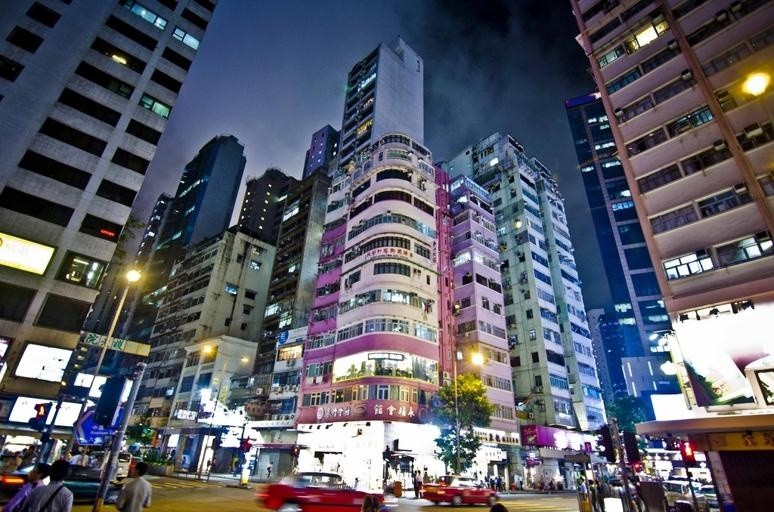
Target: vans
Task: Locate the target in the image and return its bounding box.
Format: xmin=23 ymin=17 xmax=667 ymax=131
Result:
xmin=608 ymin=479 xmax=638 ymax=500
xmin=438 ymin=474 xmax=478 ymax=487
xmin=661 ymin=480 xmax=718 ymax=512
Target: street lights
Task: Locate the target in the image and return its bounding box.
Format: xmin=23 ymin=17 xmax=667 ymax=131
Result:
xmin=453 ymin=347 xmax=485 ymax=474
xmin=198 ymin=344 xmax=249 ymax=479
xmin=63 ymin=269 xmax=141 ymax=462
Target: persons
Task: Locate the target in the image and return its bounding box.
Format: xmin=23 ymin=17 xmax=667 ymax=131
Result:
xmin=265 ymin=462 xmax=273 ymax=479
xmin=489 ymin=504 xmax=508 ymax=512
xmin=570 ymin=473 xmax=610 ymax=512
xmin=483 ymin=473 xmax=527 ymax=495
xmin=406 ymin=466 xmax=435 ymax=498
xmin=0 ymin=441 xmax=155 ymax=511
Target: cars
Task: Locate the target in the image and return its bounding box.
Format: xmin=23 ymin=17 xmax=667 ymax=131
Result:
xmin=255 ymin=471 xmax=384 ymax=512
xmin=0 ymin=462 xmax=122 ymax=505
xmin=419 ymin=483 xmax=500 ymax=506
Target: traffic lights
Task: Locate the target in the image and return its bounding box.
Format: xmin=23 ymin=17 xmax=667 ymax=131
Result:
xmin=633 ymin=462 xmax=642 ymax=472
xmin=594 ymin=428 xmax=606 ymax=458
xmin=27 ymin=403 xmax=54 ymax=430
xmin=679 ymin=439 xmax=695 ymax=464
